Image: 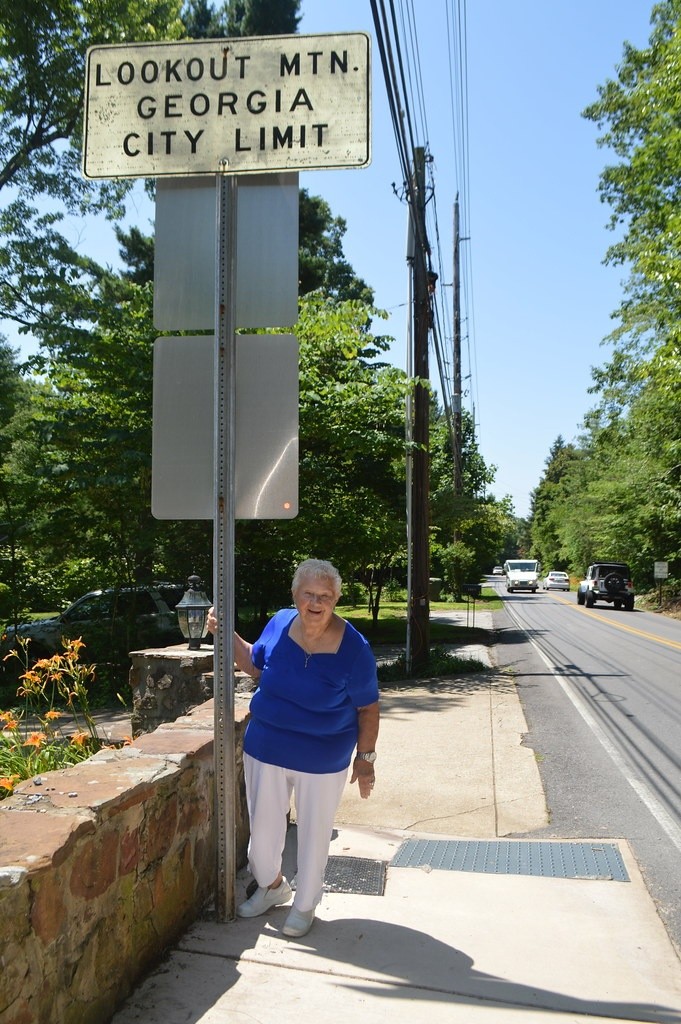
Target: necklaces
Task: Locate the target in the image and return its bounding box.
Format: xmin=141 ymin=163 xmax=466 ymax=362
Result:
xmin=299 ymin=619 xmax=331 ymax=668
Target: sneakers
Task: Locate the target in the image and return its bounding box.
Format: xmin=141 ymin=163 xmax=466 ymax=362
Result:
xmin=236 ymin=876 xmax=292 ymax=917
xmin=282 ymin=902 xmax=315 ymax=937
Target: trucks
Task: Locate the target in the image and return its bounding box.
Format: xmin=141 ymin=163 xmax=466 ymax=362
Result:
xmin=503 ymin=559 xmax=542 ymax=592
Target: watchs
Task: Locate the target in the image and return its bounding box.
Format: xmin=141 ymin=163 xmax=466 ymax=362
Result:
xmin=356 ymin=751 xmax=377 ymax=764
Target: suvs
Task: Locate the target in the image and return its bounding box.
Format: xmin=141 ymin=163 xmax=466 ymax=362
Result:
xmin=577 ymin=562 xmax=634 ymax=610
xmin=0 ymin=586 xmax=186 ymax=691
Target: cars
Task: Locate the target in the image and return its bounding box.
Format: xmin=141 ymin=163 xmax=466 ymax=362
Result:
xmin=492 ymin=566 xmax=503 ymax=575
xmin=543 ymin=571 xmax=570 ymax=591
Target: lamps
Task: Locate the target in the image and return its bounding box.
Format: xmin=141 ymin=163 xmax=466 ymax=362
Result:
xmin=175 ymin=575 xmax=212 ymax=651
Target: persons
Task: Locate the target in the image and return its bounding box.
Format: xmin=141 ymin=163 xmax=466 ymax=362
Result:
xmin=206 ymin=558 xmax=380 ymax=937
